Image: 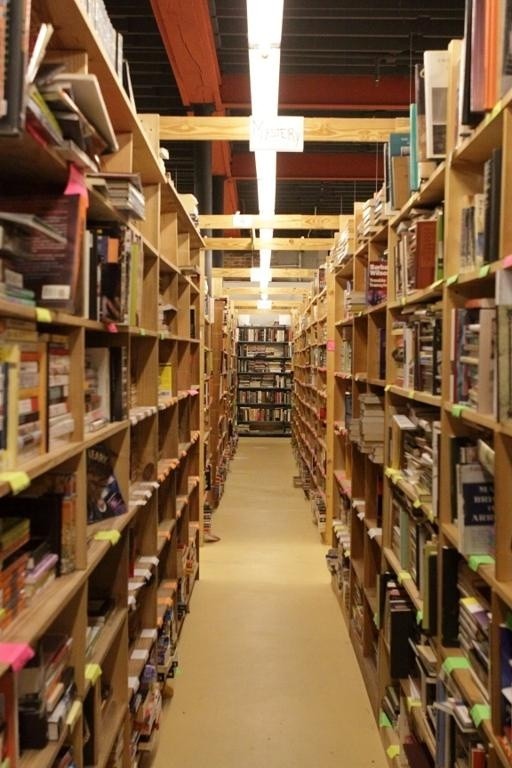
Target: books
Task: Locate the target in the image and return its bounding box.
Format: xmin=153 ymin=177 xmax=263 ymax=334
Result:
xmin=238 ymin=320 xmax=289 ymax=436
xmin=1 ymin=0 xmax=235 ymax=768
xmin=293 ymin=1 xmax=511 ymax=767
